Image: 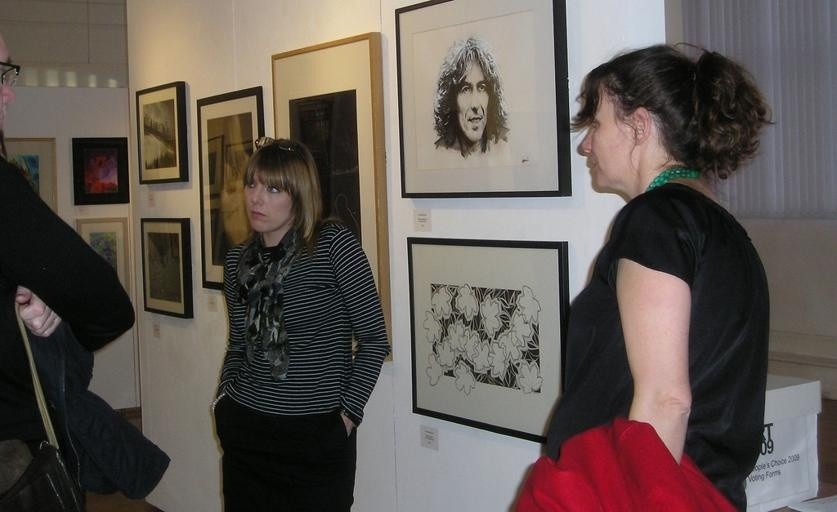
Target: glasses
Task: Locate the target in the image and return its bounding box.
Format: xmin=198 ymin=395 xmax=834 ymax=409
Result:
xmin=0 ymin=61 xmax=21 ymax=88
xmin=255 ymin=136 xmax=304 ymax=153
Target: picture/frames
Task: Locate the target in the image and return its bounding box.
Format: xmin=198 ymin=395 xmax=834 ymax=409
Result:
xmin=195 ymin=87 xmax=265 ymax=291
xmin=69 ymin=134 xmax=126 ymax=205
xmin=73 ymin=217 xmax=129 ymax=297
xmin=270 ymin=31 xmax=393 ymax=366
xmin=400 ymin=236 xmax=572 ymax=442
xmin=132 ymin=80 xmax=188 ymax=183
xmin=392 ymin=0 xmax=573 ymax=199
xmin=2 ymin=136 xmax=58 ymax=214
xmin=138 ymin=217 xmax=194 ymax=318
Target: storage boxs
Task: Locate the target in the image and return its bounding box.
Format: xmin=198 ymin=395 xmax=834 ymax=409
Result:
xmin=738 ymin=373 xmax=821 ymax=512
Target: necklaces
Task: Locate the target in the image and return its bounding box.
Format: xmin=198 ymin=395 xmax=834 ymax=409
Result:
xmin=642 ymin=161 xmax=707 ymax=191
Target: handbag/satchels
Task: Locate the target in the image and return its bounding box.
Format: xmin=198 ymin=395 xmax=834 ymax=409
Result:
xmin=0 ymin=440 xmax=86 ymax=511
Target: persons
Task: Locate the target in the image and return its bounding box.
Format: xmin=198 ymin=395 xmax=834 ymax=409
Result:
xmin=0 ymin=34 xmax=136 ymax=509
xmin=428 ymin=30 xmax=510 ymax=161
xmin=517 ymin=40 xmax=776 ymax=511
xmin=205 ymin=135 xmax=392 ymax=510
